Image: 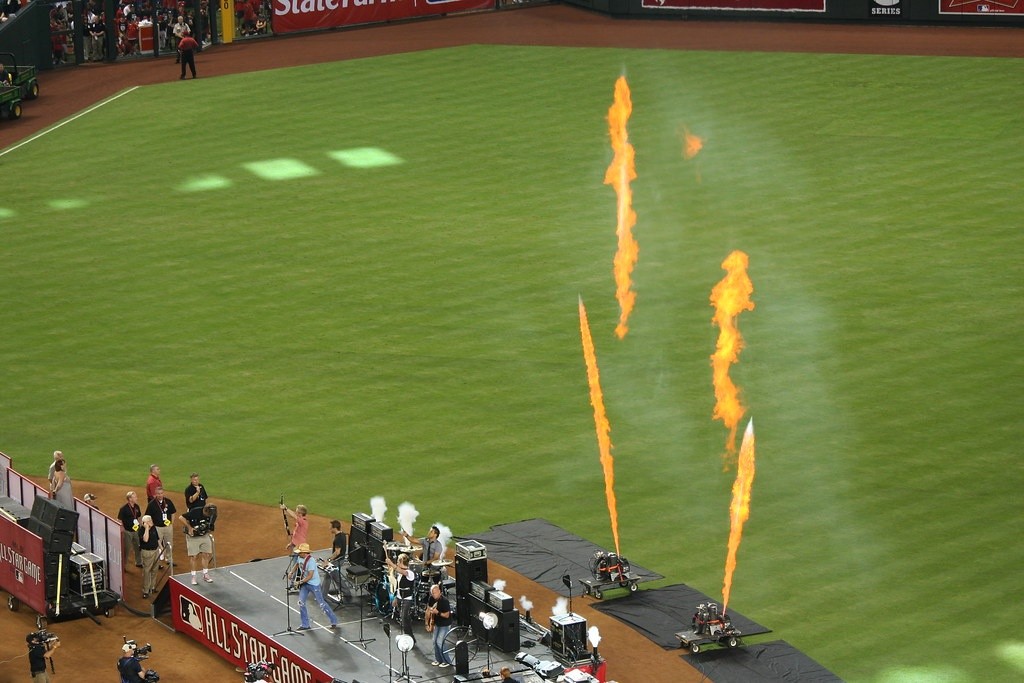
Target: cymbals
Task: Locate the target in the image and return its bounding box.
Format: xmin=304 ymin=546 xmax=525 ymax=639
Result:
xmin=400 ymin=546 xmax=423 ymax=552
xmin=431 ymin=559 xmax=454 ymax=567
xmin=409 ymin=561 xmax=424 ymax=565
xmin=422 ymin=570 xmax=441 ymax=577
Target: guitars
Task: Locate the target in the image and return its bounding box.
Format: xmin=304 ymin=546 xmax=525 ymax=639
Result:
xmin=382 ymin=540 xmax=396 ymax=594
xmin=425 ymin=601 xmax=438 ymax=633
xmin=293 ymin=576 xmax=302 ymax=591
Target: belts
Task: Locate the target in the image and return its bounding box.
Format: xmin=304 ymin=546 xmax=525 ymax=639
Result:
xmin=35 ymin=670 xmax=46 ymax=674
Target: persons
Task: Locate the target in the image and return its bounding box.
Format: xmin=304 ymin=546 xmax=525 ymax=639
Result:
xmin=0 ymin=63 xmax=9 ymax=86
xmin=83 ymin=494 xmax=99 ymax=510
xmin=0 ymin=0 xmax=273 ymax=64
xmin=138 ymin=515 xmax=165 ymax=599
xmin=425 ymin=585 xmax=452 ymax=667
xmin=401 ymin=526 xmax=442 ymax=568
xmin=385 ymin=553 xmax=417 ymax=645
xmin=115 ymin=464 xmax=177 ymax=572
xmin=48 ymin=450 xmax=74 ymax=512
xmin=499 ymin=667 xmax=520 ymax=683
xmin=116 ymin=644 xmax=146 ymax=683
xmin=279 ymin=503 xmax=309 ymax=590
xmin=183 ymin=472 xmax=209 ymax=513
xmin=321 ymin=519 xmax=352 ymax=600
xmin=25 ymin=632 xmax=60 ymax=683
xmin=179 ymin=503 xmax=218 ymax=585
xmin=288 ymin=542 xmax=338 ymax=630
xmin=179 ymin=32 xmax=199 ymax=79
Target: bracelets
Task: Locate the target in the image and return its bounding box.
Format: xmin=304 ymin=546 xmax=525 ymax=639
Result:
xmin=406 ymin=534 xmax=409 ymax=538
xmin=290 ymin=572 xmax=293 ymax=574
xmin=53 ymin=646 xmax=57 ymax=649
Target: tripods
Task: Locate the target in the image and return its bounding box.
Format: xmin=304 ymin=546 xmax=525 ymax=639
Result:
xmin=330 ymin=543 xmax=362 ymax=612
xmin=379 ymin=624 xmax=422 ymax=683
xmin=272 ymin=556 xmax=305 ymax=637
xmin=344 ymin=585 xmax=376 ymax=650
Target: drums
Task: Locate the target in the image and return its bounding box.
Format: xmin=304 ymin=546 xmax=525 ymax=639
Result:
xmin=375 ymin=582 xmax=397 ymax=615
xmin=418 ymin=582 xmax=429 ymax=593
xmin=383 ymin=543 xmax=409 ymax=559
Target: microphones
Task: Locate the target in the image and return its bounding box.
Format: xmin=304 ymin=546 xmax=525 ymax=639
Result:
xmin=290 ymin=554 xmax=300 ymax=557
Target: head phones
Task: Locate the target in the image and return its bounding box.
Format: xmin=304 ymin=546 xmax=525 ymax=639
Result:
xmin=145 ymin=669 xmax=159 ymax=683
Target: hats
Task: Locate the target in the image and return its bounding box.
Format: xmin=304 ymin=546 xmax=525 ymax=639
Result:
xmin=26 ymin=632 xmax=38 ymax=643
xmin=293 ymin=543 xmax=311 ymax=553
xmin=122 ymin=644 xmax=136 ymax=653
xmin=84 ymin=493 xmax=95 ymax=502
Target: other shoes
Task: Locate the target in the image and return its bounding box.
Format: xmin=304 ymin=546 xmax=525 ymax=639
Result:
xmin=158 ymin=565 xmax=164 ymax=569
xmin=135 ymin=563 xmax=144 ymax=568
xmin=203 ymin=573 xmax=214 ymax=583
xmin=191 ymin=575 xmax=198 ymax=585
xmin=285 ymin=587 xmax=294 ymax=592
xmin=330 ymin=625 xmax=336 ymax=629
xmin=143 ymin=592 xmax=149 ymax=598
xmin=167 ymin=561 xmax=176 ymax=566
xmin=297 ymin=626 xmax=310 ymax=630
xmin=438 ymin=662 xmax=449 ymax=667
xmin=431 ymin=661 xmax=440 ymax=666
xmin=152 ymin=589 xmax=158 ymax=593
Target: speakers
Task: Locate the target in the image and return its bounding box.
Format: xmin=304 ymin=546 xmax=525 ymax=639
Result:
xmin=455 ymin=556 xmax=520 ymax=653
xmin=26 ymin=495 xmax=80 ymax=599
xmin=348 ymin=525 xmax=386 ymax=579
